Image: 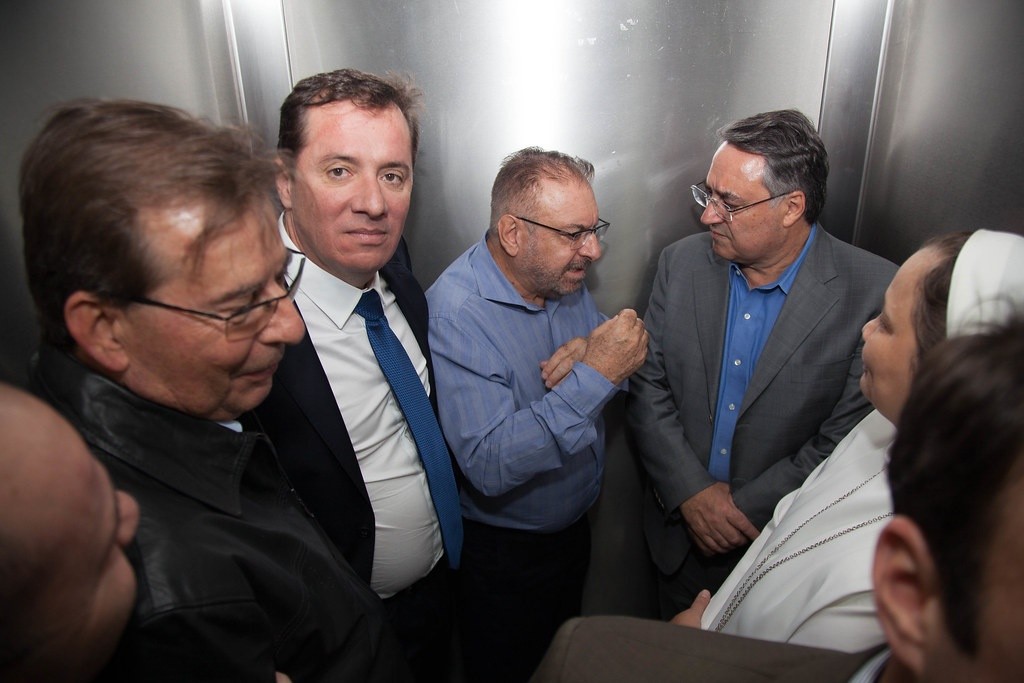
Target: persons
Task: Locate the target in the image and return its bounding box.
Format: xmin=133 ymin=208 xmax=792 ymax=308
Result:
xmin=0 ymin=383 xmax=142 ymax=682
xmin=629 ymin=109 xmax=900 ymax=622
xmin=425 ymin=146 xmax=651 ymax=683
xmin=670 ymin=227 xmax=1024 ymax=653
xmin=536 ymin=293 xmax=1024 ymax=683
xmin=19 ymin=98 xmax=412 ymax=683
xmin=235 ymin=67 xmax=465 ymax=683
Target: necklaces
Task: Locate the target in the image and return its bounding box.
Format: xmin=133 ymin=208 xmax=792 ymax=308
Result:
xmin=715 ymin=459 xmax=899 ymax=634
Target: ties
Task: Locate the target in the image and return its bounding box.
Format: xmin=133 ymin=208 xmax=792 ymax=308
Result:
xmin=354 ymin=287 xmax=465 ymax=573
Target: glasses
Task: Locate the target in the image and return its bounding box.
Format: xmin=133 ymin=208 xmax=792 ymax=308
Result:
xmin=516 ymin=217 xmax=611 ymax=250
xmin=98 ymin=248 xmax=307 ymax=343
xmin=691 ymin=179 xmax=793 ymax=222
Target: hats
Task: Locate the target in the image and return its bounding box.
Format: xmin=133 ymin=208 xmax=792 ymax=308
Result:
xmin=946 ymin=228 xmax=1023 ymax=337
